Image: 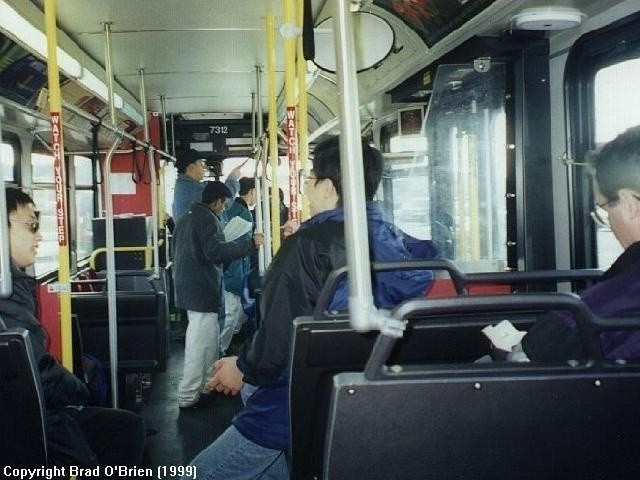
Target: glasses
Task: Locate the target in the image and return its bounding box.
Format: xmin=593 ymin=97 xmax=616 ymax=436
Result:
xmin=590 ymin=193 xmax=640 ymax=228
xmin=7 ymin=218 xmax=40 ymax=233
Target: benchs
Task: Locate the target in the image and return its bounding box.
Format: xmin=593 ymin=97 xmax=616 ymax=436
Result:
xmin=288 ymin=257 xmax=640 ymax=480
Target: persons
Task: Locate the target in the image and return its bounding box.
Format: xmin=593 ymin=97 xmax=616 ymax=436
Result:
xmin=171 ymin=147 xmax=244 ymax=226
xmin=174 ymin=135 xmax=444 ymax=479
xmin=165 ymin=179 xmax=266 ymax=410
xmin=1 ymin=186 xmax=149 ymax=477
xmin=265 ymin=185 xmax=290 ymax=230
xmin=466 ymin=124 xmax=640 ymax=369
xmin=213 ymin=176 xmax=263 ymax=357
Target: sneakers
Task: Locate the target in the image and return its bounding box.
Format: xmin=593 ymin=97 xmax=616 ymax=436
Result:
xmin=178 ymin=393 xmax=211 ymax=412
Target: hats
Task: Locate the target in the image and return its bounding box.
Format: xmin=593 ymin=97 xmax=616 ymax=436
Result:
xmin=174 ymin=144 xmax=202 ymax=172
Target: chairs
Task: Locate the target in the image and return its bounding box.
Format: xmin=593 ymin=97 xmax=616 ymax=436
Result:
xmin=0 ymin=270 xmax=168 ymax=480
